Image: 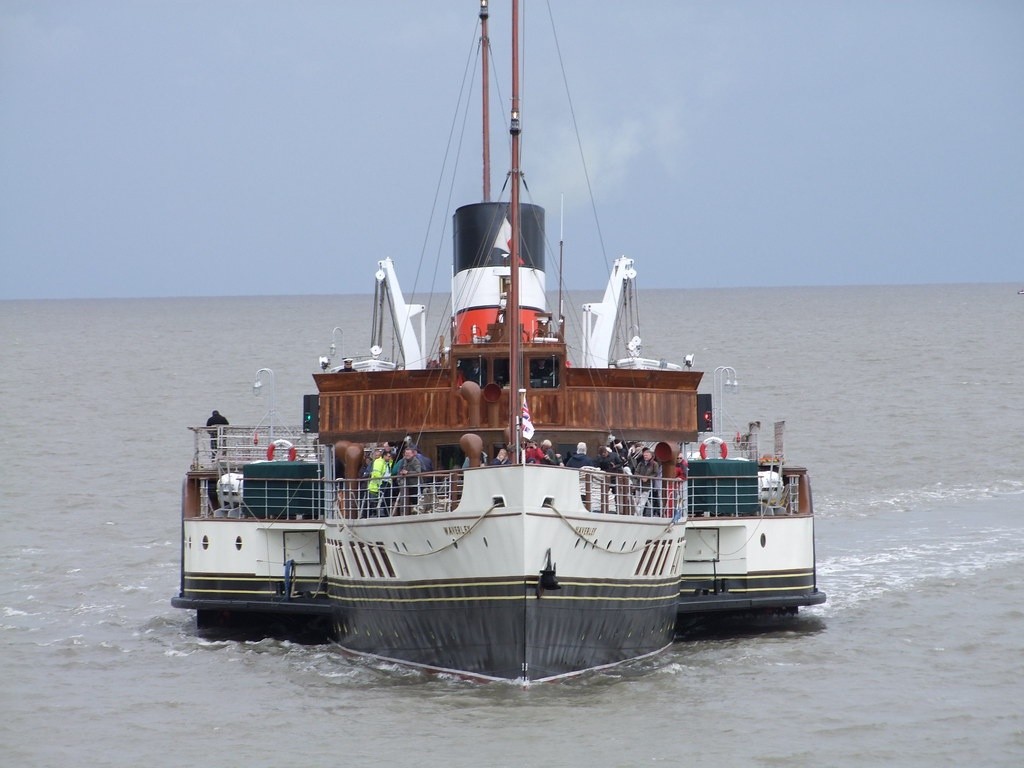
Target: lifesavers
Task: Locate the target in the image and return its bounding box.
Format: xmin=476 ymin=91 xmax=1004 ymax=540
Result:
xmin=700 ymin=437 xmax=729 ymax=461
xmin=267 ymin=440 xmax=296 ymax=462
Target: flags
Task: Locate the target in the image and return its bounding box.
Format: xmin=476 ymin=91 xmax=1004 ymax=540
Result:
xmin=493 ymin=217 xmax=524 ymax=265
xmin=521 ymin=402 xmax=535 ymax=441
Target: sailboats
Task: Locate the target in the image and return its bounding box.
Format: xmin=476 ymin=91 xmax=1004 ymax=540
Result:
xmin=167 ymin=1 xmax=827 ymax=689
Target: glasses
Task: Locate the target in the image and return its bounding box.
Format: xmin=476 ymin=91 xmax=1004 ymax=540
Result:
xmin=388 ymin=456 xmax=392 ymax=458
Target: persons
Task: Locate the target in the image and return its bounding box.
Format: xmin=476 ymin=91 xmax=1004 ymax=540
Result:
xmin=357 ymin=439 xmax=687 ymax=519
xmin=337 ymin=358 xmax=358 ymax=372
xmin=466 ymin=359 xmax=551 ymax=388
